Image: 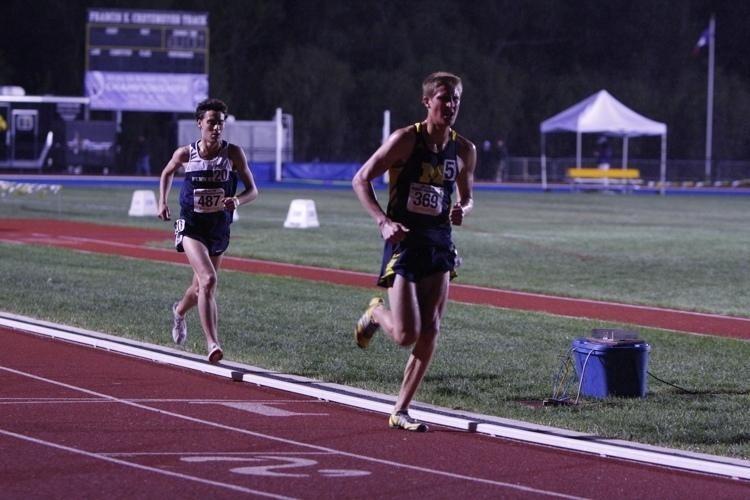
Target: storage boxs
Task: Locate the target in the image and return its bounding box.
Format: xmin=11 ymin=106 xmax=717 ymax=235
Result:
xmin=572 ymin=338 xmax=651 ymax=400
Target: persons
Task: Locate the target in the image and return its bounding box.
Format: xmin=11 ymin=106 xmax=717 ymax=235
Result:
xmin=113 ymin=123 xmax=128 ymax=177
xmin=494 ymin=140 xmax=510 ymax=184
xmin=135 ymin=132 xmax=159 ymax=177
xmin=349 ymin=68 xmax=477 ymax=431
xmin=159 ymin=98 xmax=260 ymax=365
xmin=482 ymin=141 xmax=494 ymax=181
xmin=593 ymin=135 xmax=612 ymax=171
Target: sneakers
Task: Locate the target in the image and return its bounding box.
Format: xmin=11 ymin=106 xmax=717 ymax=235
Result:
xmin=173 ymin=303 xmax=186 ymax=344
xmin=208 ymin=343 xmax=223 ymax=363
xmin=355 ymin=297 xmax=384 ymax=348
xmin=388 ymin=413 xmax=428 ymax=432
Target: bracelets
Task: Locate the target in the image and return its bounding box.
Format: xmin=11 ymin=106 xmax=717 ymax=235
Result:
xmin=233 ymin=196 xmax=241 ymax=208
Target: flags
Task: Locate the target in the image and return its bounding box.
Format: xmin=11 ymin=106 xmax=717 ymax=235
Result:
xmin=688 ymin=14 xmax=716 ymax=55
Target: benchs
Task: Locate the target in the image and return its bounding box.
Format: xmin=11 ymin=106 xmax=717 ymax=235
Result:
xmin=564 ymin=168 xmax=644 ymax=184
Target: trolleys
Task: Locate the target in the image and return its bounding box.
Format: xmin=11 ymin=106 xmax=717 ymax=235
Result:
xmin=1 ymin=130 xmax=54 ymax=174
